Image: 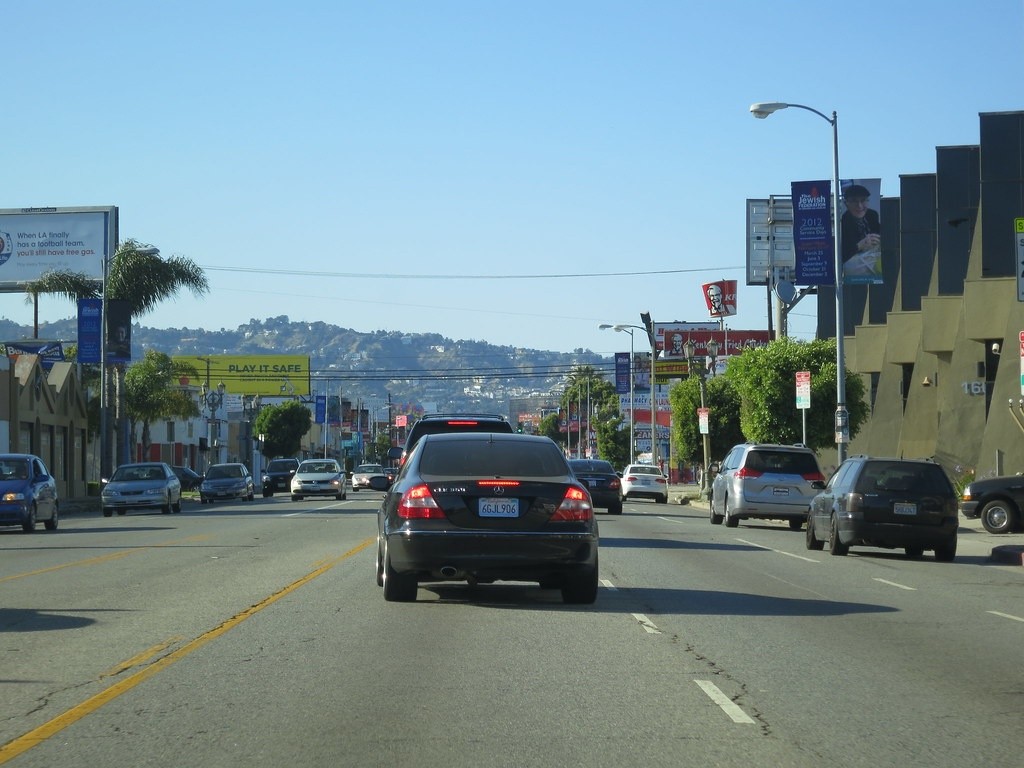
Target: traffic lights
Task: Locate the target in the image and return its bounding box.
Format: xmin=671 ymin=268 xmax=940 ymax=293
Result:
xmin=517 ymin=421 xmax=523 ymax=434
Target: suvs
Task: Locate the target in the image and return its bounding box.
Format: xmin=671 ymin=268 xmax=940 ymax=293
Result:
xmin=708 ymin=441 xmax=827 ymax=529
xmin=387 ymin=412 xmax=516 ymax=475
xmin=259 ymin=457 xmax=301 ymax=497
xmin=805 ymin=453 xmax=958 ymax=561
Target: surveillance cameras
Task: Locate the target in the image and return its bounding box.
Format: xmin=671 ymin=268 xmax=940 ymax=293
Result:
xmin=992 ymin=343 xmax=999 ymax=354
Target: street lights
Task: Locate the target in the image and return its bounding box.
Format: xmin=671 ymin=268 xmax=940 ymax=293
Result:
xmin=355 ymin=393 xmax=379 ymax=453
xmin=100 ymin=247 xmax=161 ymax=486
xmin=611 ymin=324 xmax=657 ymax=465
xmin=598 ymin=324 xmax=635 ymax=464
xmin=201 ymin=380 xmax=226 ymax=467
xmin=371 ymin=405 xmax=391 ymax=463
xmin=681 ymin=331 xmax=719 ymax=502
xmin=240 ymin=393 xmax=264 ymax=465
xmin=338 ymin=381 xmax=361 ymax=468
xmin=748 ymin=100 xmax=848 ymax=467
xmin=547 ymin=369 xmax=590 ymax=458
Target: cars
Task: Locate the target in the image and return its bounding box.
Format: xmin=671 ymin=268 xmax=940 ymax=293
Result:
xmin=99 ymin=462 xmax=182 ymax=518
xmin=618 ymin=464 xmax=669 ymax=503
xmin=565 ymin=458 xmax=624 ymax=515
xmin=198 ymin=463 xmax=257 ymax=505
xmin=383 ymin=468 xmax=400 ymax=484
xmin=349 ymin=464 xmax=386 ymax=493
xmin=289 ymin=458 xmax=347 ymax=501
xmin=375 ymin=432 xmax=600 ymax=602
xmin=960 ymin=469 xmax=1024 ymax=535
xmin=171 ymin=465 xmax=203 ymax=491
xmin=0 ymin=453 xmax=59 ymax=533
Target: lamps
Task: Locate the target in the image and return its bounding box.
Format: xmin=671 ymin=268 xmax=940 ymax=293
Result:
xmin=977 ymin=361 xmax=985 ymax=377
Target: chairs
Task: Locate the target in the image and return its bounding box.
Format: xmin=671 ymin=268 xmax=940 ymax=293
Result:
xmin=886 ymin=478 xmax=902 ymax=488
xmin=862 ymin=477 xmax=877 ymax=489
xmin=631 ymin=468 xmax=638 ymax=473
xmin=649 ymin=469 xmax=657 ymax=474
